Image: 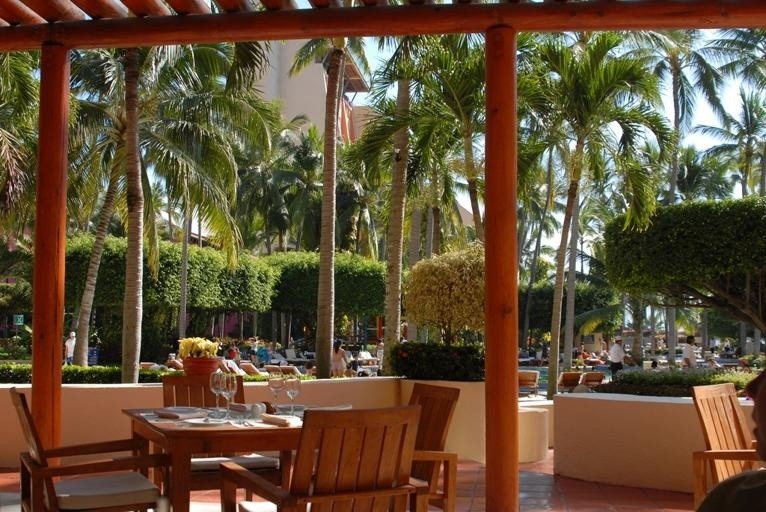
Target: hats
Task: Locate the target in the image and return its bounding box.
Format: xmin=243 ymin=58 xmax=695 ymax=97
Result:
xmin=615 ymin=336 xmax=623 ymax=340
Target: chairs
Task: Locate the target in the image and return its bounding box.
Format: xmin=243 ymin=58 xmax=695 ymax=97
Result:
xmin=9 ymin=387 xmax=170 ymax=512
xmin=219 ymin=403 xmax=422 ymax=512
xmin=167 ymin=336 xmax=381 ymax=377
xmin=691 ymin=383 xmax=761 ymax=512
xmin=153 ymin=375 xmax=292 ymax=512
xmin=308 ymin=382 xmax=460 ymax=512
xmin=518 ymin=350 xmax=604 ymax=397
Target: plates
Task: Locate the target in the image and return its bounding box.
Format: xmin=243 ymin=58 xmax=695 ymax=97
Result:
xmin=182 ymin=417 xmax=228 ymax=426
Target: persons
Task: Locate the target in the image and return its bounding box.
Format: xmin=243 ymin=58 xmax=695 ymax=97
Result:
xmin=575 ymin=335 xmax=742 ymax=380
xmin=332 ymin=335 xmax=408 ymax=377
xmin=697 ymin=367 xmax=766 ymax=512
xmin=539 ymin=339 xmax=549 ymax=367
xmin=224 ymin=336 xmax=295 ymax=364
xmin=63 ymin=331 xmax=76 ymax=365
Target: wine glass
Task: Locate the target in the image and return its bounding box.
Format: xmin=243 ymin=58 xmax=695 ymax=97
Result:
xmin=268 ymin=371 xmax=285 ymax=413
xmin=219 ymin=374 xmax=238 ymax=420
xmin=209 ymin=371 xmax=226 ymax=418
xmin=285 ymin=373 xmax=301 ymax=421
